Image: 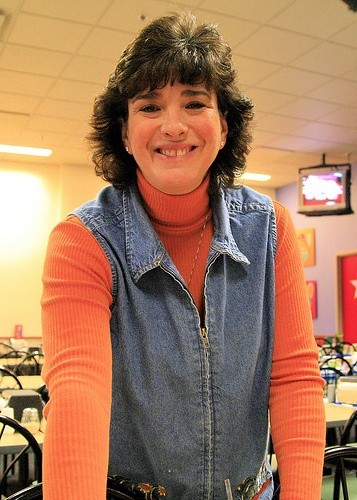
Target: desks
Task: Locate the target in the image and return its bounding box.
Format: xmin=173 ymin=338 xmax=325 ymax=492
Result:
xmin=0 ymin=338 xmax=357 ymax=500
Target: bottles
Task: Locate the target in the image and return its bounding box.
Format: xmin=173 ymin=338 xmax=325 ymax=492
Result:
xmin=328 ymin=380 xmax=334 ymax=402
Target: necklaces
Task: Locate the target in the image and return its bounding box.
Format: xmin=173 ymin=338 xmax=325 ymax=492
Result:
xmin=187 ymin=215 xmax=208 ymax=288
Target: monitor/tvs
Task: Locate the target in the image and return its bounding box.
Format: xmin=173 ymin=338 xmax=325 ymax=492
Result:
xmin=298 ymin=164 xmax=350 ymax=214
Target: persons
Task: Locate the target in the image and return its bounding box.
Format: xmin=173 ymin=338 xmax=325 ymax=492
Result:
xmin=40 ymin=10 xmax=327 ymax=500
